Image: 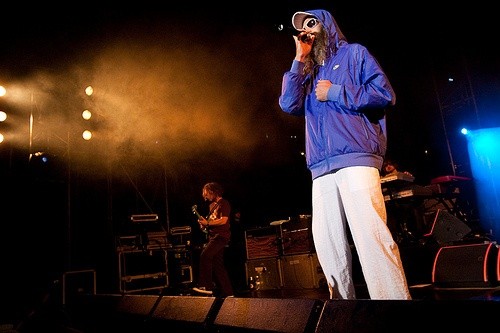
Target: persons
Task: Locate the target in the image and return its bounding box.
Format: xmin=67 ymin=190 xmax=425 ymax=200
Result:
xmin=278 ymin=10 xmax=416 ymax=300
xmin=192 ymin=182 xmax=244 ymax=299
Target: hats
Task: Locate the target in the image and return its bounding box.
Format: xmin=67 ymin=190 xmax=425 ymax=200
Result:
xmin=291 ymin=11 xmax=314 ymax=32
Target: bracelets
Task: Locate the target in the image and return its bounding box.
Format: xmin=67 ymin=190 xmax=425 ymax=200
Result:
xmin=207 ymin=221 xmax=209 ymax=227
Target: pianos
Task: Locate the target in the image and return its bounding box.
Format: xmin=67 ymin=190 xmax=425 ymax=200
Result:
xmin=380 ymin=173 xmax=461 ymax=243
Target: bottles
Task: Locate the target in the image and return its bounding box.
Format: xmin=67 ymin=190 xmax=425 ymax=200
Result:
xmin=255 ymin=276 xmax=261 ymax=290
xmin=249 ymin=277 xmax=255 ymax=289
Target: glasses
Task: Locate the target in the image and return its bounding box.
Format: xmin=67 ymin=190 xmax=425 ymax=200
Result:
xmin=303 ymin=18 xmax=317 ymax=32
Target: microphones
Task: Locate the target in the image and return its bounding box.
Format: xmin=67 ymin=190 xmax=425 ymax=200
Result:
xmin=278 ymin=24 xmax=312 ymax=43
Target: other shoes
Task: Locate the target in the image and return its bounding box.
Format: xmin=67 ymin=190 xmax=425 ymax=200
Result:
xmin=192 ymin=287 xmax=213 ymax=294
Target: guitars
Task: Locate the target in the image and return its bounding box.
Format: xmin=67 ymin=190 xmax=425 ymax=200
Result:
xmin=192 ymin=205 xmax=208 ymax=234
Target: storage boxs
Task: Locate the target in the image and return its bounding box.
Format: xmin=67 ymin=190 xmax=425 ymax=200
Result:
xmin=170 ymin=226 xmax=191 ymax=235
xmin=118 ymin=249 xmax=170 ymax=294
xmin=63 ymin=269 xmax=97 ymax=303
xmin=131 ymin=214 xmax=159 ymax=221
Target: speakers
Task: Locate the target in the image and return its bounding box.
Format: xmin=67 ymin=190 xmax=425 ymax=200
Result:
xmin=428 ymin=209 xmax=472 ymax=242
xmin=245 ymin=218 xmax=326 ymax=290
xmin=91 ymin=294 xmax=500 ymax=333
xmin=432 ymin=243 xmax=498 ymax=288
